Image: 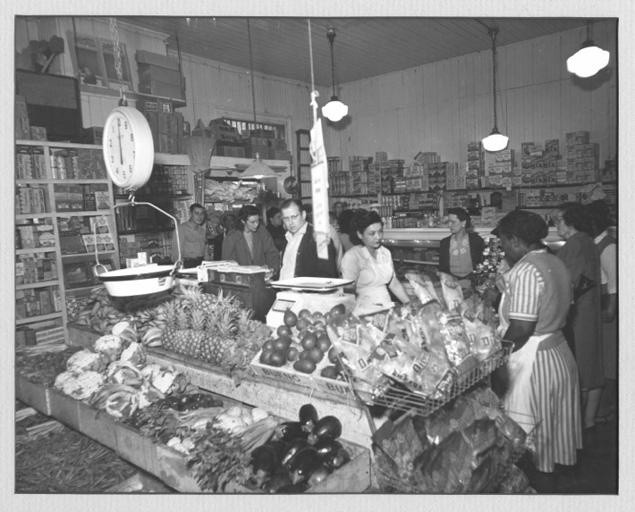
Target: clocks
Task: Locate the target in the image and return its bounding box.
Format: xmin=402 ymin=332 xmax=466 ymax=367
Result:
xmin=100 ymin=96 xmax=155 ymax=191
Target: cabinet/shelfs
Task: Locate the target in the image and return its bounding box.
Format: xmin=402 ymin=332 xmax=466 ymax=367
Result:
xmin=349 ymin=308 xmax=538 ymax=495
xmin=381 ymin=189 xmax=443 ymax=278
xmin=199 ymin=157 xmax=289 ymax=231
xmin=443 ymin=186 xmax=519 ymax=258
xmin=115 ymin=153 xmax=198 ymax=264
xmin=326 ymin=194 xmax=378 ymax=219
xmin=14 ymin=327 xmax=375 ymax=496
xmin=14 ymin=137 xmax=119 ymax=360
xmin=520 ymin=183 xmax=619 ymax=253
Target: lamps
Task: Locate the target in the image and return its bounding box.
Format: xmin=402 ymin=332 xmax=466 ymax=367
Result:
xmin=319 ymin=33 xmax=350 ymax=122
xmin=237 ymin=21 xmax=282 ymax=183
xmin=565 ymin=17 xmax=610 ymax=80
xmin=478 ymin=33 xmax=512 ymax=153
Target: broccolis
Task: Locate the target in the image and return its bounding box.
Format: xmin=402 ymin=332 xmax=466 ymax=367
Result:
xmin=54 ymin=321 xmax=175 ymax=422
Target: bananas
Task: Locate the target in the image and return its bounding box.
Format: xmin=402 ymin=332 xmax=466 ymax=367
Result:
xmin=74 ymin=288 xmax=160 ymax=351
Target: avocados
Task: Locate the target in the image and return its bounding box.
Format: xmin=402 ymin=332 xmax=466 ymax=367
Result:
xmin=259 ymin=303 xmax=347 ymax=376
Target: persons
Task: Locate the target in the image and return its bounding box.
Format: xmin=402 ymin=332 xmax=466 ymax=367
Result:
xmin=438 ymin=199 xmax=616 ymax=474
xmin=171 ymin=197 xmax=411 ymax=307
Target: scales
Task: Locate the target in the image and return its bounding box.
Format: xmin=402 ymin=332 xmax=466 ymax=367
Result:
xmin=267 ymin=276 xmax=356 ymax=327
xmin=93 ymin=98 xmax=182 ymax=298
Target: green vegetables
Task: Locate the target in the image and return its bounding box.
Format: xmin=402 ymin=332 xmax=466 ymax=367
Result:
xmin=184 ymin=417 xmax=279 ymax=495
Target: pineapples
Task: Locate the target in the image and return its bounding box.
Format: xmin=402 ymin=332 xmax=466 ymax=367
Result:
xmin=160 ymin=285 xmax=265 ymax=371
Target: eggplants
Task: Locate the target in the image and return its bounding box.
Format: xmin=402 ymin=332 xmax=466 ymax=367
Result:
xmin=249 ymin=404 xmax=349 ymax=493
